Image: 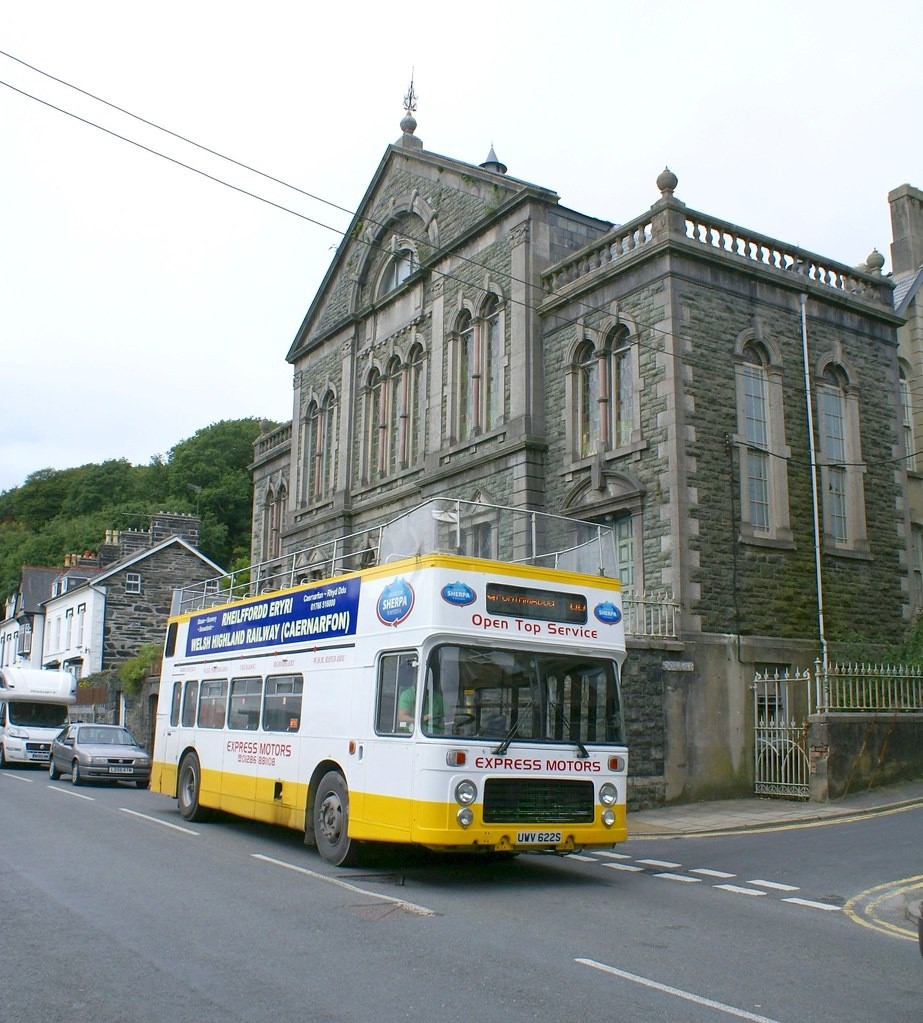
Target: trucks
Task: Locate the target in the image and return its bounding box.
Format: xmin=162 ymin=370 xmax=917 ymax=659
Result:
xmin=0 ymin=666 xmax=77 ymax=768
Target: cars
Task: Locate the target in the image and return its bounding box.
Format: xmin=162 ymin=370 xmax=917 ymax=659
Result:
xmin=48 ymin=722 xmax=152 ymax=788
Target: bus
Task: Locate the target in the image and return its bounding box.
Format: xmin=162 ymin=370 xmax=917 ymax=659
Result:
xmin=148 ymin=497 xmax=629 ymax=866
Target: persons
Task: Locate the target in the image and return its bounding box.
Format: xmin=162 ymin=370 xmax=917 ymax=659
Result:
xmin=398 ymin=671 xmax=444 ymax=734
